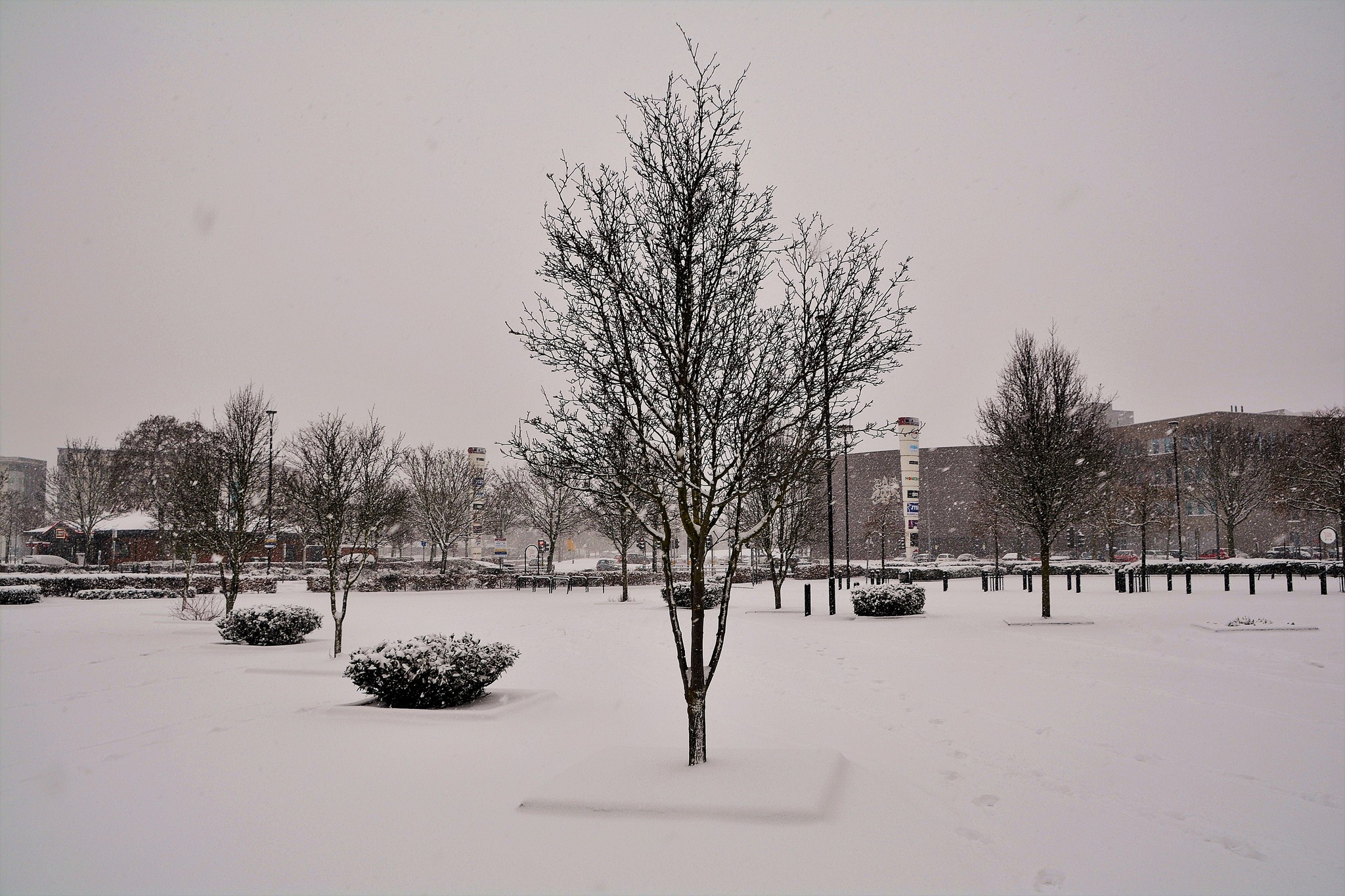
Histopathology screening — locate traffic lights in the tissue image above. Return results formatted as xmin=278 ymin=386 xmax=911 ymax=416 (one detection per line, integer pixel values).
xmin=706 ymin=534 xmax=713 ymax=550
xmin=637 ymin=537 xmax=645 ymax=551
xmin=538 ymin=539 xmax=549 ymax=554
xmin=657 ymin=537 xmax=662 ymax=550
xmin=728 ymin=539 xmax=734 ymax=548
xmin=688 ymin=536 xmax=693 ymax=548
xmin=672 ymin=538 xmax=680 ymax=549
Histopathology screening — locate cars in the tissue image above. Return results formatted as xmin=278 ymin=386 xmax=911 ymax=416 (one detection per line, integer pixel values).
xmin=895 ymin=545 xmax=1294 ymax=564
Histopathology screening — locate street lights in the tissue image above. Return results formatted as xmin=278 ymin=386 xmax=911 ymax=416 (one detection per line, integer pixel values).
xmin=263 ymin=409 xmax=276 ymax=575
xmin=835 ymin=424 xmax=856 ymax=588
xmin=1167 ymin=421 xmax=1184 ymax=562
